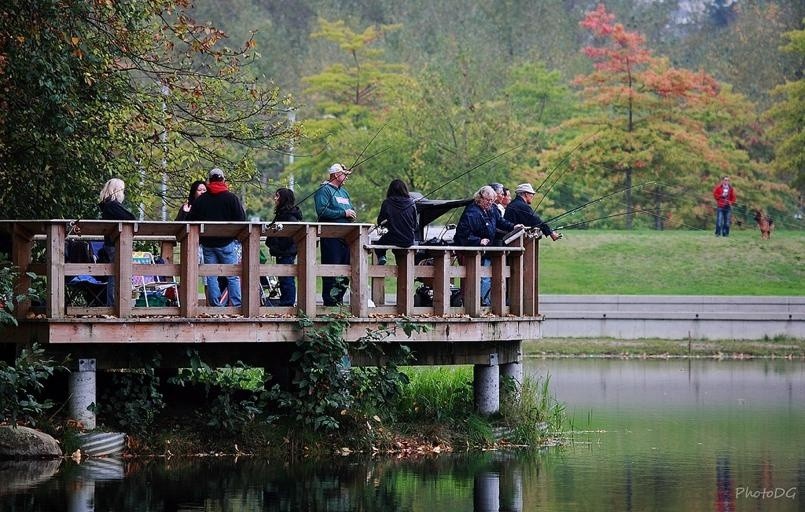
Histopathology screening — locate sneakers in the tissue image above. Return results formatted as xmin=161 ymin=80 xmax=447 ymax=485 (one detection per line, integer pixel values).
xmin=377 ymin=256 xmax=387 ymax=265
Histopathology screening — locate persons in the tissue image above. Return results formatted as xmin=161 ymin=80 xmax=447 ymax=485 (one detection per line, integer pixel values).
xmin=713 ymin=174 xmax=737 ymax=238
xmin=453 ymin=182 xmax=561 ymax=306
xmin=711 ymin=452 xmax=742 ymax=512
xmin=314 ymin=162 xmax=358 ymax=307
xmin=264 ymin=186 xmax=304 ymax=307
xmin=97 ymin=177 xmax=138 ymax=307
xmin=177 ymin=179 xmax=209 ymax=307
xmin=189 ymin=166 xmax=246 ymax=307
xmin=373 ymin=178 xmax=417 ymax=265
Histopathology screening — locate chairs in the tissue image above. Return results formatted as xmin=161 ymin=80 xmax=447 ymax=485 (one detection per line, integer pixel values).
xmin=61 ymin=239 xmax=181 ymax=309
xmin=258 ymin=248 xmax=281 ymax=306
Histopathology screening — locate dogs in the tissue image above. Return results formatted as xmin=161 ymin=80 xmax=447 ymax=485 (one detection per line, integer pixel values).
xmin=754 ymin=209 xmax=776 ymax=239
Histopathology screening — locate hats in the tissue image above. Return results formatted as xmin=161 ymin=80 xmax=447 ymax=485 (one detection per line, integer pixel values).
xmin=514 ymin=182 xmax=537 ymax=194
xmin=329 ymin=163 xmax=352 ymax=175
xmin=209 ymin=168 xmax=225 ymax=179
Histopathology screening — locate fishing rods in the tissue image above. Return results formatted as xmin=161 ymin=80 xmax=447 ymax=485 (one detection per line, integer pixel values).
xmin=520 ymin=129 xmax=675 ymax=233
xmin=270 ymin=122 xmax=384 ymax=298
xmin=367 ymin=143 xmax=527 ymax=235
xmin=272 ymin=146 xmax=393 ymax=224
